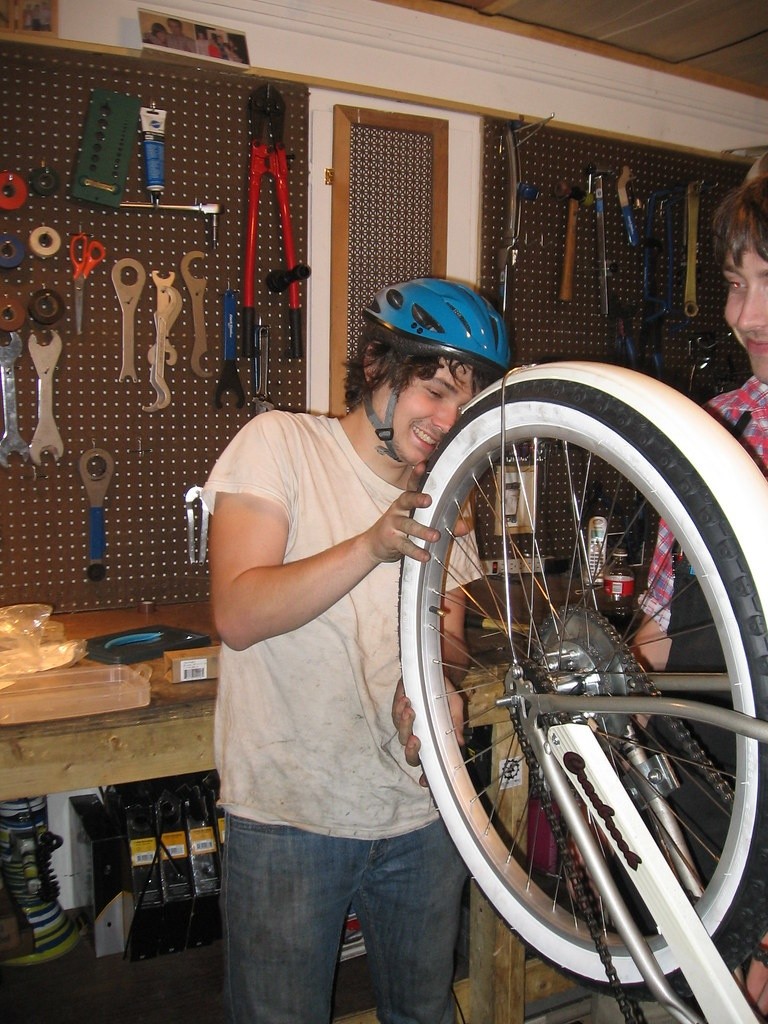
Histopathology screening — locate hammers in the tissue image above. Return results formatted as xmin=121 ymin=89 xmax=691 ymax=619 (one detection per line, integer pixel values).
xmin=551 ymin=181 xmax=594 ymax=302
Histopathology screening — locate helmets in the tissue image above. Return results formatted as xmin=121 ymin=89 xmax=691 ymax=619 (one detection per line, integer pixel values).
xmin=361 ymin=277 xmax=510 ymax=378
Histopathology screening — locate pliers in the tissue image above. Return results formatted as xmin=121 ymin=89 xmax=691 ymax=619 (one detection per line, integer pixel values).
xmin=237 ymin=83 xmax=305 ymax=359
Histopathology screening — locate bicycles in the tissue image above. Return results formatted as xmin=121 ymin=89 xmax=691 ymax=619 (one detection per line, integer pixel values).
xmin=395 ymin=357 xmax=767 ymax=1024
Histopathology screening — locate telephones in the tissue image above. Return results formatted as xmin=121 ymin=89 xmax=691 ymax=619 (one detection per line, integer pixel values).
xmin=581 ymin=514 xmax=608 ymax=585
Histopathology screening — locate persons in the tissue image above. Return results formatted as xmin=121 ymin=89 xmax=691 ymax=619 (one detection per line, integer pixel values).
xmin=200 ymin=276 xmax=508 ymax=1024
xmin=638 ymin=177 xmax=768 ymax=1024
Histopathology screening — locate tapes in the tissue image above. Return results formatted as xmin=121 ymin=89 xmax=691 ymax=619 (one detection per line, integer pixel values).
xmin=0 ymin=299 xmax=25 ymax=331
xmin=29 ymin=226 xmax=62 ymax=259
xmin=0 ymin=172 xmax=27 ymax=210
xmin=0 ymin=234 xmax=25 ymax=269
xmin=30 ymin=166 xmax=60 ymax=195
xmin=28 ymin=288 xmax=64 ymax=326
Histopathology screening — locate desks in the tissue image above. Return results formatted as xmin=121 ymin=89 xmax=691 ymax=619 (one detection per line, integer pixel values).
xmin=0 ymin=601 xmax=530 ymax=1024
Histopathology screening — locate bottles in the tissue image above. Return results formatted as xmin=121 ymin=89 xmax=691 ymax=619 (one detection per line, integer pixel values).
xmin=603 ymin=548 xmax=634 ymax=631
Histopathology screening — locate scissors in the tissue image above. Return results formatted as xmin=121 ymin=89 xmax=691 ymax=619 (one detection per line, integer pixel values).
xmin=69 ymin=232 xmax=107 ymax=335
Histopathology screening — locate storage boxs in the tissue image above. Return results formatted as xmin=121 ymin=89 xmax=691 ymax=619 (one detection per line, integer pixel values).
xmin=0 ymin=663 xmax=153 ymax=725
xmin=202 ymin=789 xmax=226 ymax=861
xmin=179 ymin=792 xmax=222 ymax=950
xmin=150 ymin=792 xmax=194 ymax=955
xmin=103 ymin=795 xmax=164 ymax=963
xmin=164 ymin=647 xmax=221 ymax=683
xmin=69 ymin=794 xmax=125 ymax=959
